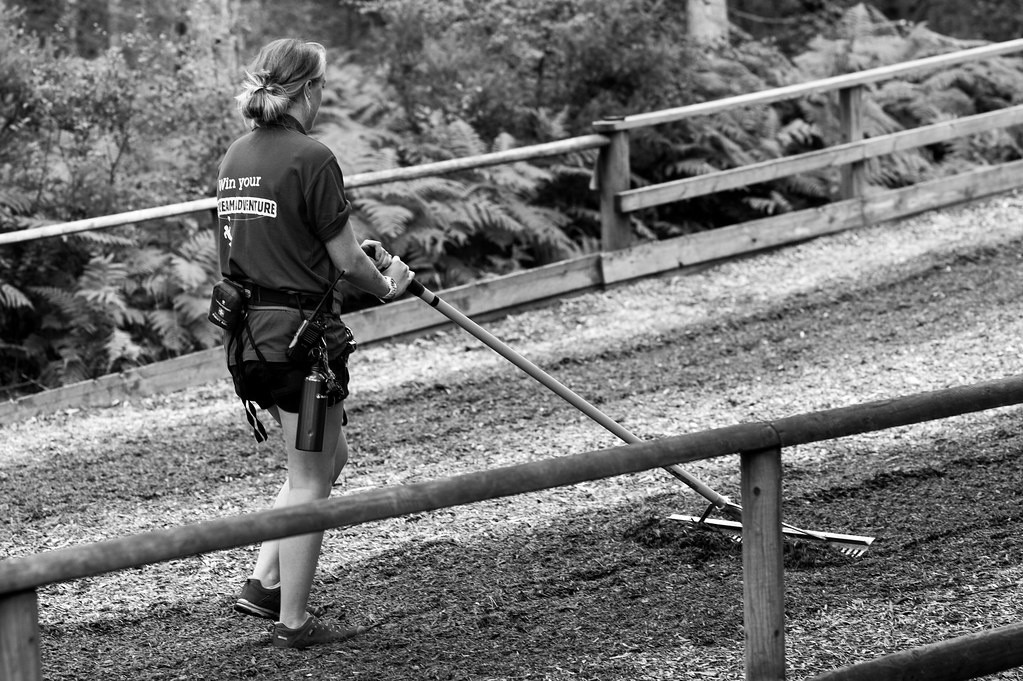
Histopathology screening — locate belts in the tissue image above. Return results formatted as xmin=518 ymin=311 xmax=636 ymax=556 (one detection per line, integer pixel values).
xmin=245 ymin=286 xmax=341 ymax=316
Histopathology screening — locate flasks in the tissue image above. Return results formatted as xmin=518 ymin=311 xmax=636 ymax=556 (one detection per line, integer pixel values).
xmin=295 ymin=348 xmax=328 ymax=452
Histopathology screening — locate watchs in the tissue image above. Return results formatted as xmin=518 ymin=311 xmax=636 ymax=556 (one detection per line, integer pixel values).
xmin=379 ymin=275 xmax=396 ymax=304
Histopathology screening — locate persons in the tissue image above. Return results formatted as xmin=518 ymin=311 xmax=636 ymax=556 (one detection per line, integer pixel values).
xmin=216 ymin=38 xmax=414 ymax=650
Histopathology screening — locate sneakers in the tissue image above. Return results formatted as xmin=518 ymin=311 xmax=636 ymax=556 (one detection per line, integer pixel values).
xmin=275 ymin=614 xmax=355 ymax=650
xmin=234 ymin=578 xmax=283 ymax=619
